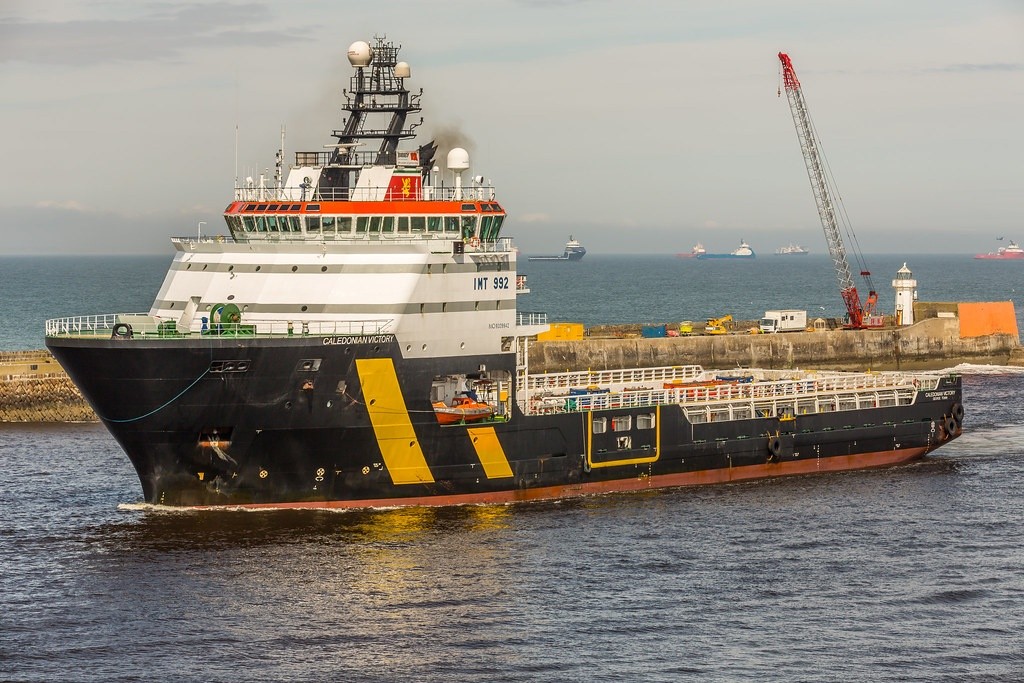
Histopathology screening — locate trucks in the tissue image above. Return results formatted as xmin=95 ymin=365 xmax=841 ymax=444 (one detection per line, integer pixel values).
xmin=757 ymin=309 xmax=807 ymax=333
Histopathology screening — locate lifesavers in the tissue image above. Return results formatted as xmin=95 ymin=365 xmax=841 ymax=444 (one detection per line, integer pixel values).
xmin=517 ymin=277 xmax=524 ymax=288
xmin=768 ymin=437 xmax=784 ymax=457
xmin=470 ymin=236 xmax=480 ymax=247
xmin=945 ymin=418 xmax=957 ymax=437
xmin=113 ymin=322 xmax=130 ymax=338
xmin=952 ymin=403 xmax=964 ymax=422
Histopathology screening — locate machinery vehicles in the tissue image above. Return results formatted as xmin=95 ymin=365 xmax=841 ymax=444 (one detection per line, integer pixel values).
xmin=775 ymin=50 xmax=885 ymax=330
xmin=702 ymin=315 xmax=735 ymax=335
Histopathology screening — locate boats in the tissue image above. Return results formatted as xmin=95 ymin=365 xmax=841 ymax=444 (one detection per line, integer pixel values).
xmin=44 ymin=36 xmax=965 ymax=515
xmin=527 ymin=235 xmax=586 ymax=261
xmin=697 ymin=238 xmax=756 ymax=260
xmin=436 ymin=398 xmax=492 ymax=425
xmin=676 ymin=247 xmax=705 ymax=259
xmin=974 ymin=237 xmax=1024 ymax=261
xmin=774 ymin=244 xmax=808 ymax=256
